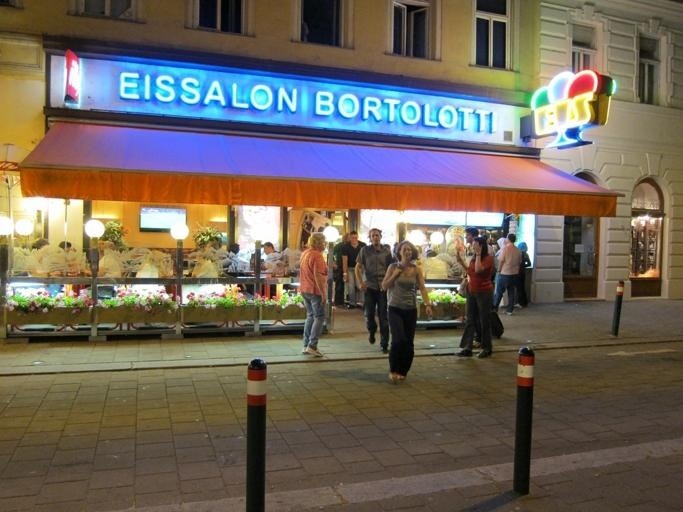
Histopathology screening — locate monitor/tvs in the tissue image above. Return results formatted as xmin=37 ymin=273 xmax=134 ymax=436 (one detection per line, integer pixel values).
xmin=139 ymin=205 xmax=186 ymax=232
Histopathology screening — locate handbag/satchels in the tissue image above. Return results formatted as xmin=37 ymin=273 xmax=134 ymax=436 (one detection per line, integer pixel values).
xmin=458 ymin=277 xmax=469 ymax=298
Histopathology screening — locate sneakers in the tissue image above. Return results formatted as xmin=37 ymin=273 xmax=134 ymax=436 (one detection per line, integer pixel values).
xmin=369 ymin=331 xmax=376 ymax=344
xmin=380 ymin=346 xmax=388 ymax=353
xmin=499 ymin=303 xmax=522 ymax=315
xmin=302 ymin=344 xmax=323 ymax=357
xmin=456 ymin=339 xmax=492 ymax=358
xmin=389 ymin=373 xmax=404 ymax=382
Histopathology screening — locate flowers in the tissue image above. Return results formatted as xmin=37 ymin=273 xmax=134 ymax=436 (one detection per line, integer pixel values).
xmin=192 ymin=223 xmax=222 ymax=246
xmin=99 ymin=221 xmax=128 ymax=243
xmin=416 ymin=288 xmax=466 ymax=307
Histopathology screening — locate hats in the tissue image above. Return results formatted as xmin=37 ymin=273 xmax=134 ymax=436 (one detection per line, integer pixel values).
xmin=261 ymin=242 xmax=273 ymax=247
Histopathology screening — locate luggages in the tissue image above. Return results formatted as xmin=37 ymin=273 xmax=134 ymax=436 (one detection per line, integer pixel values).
xmin=488 ymin=310 xmax=504 ymax=339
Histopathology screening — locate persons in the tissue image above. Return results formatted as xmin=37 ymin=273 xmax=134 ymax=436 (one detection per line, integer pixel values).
xmin=26 ymin=238 xmax=144 ymax=279
xmin=465 ymin=227 xmax=495 ymax=348
xmin=456 ymin=238 xmax=494 ymax=358
xmin=381 ymin=240 xmax=433 ymax=383
xmin=189 ymin=231 xmax=531 ymax=315
xmin=354 ymin=228 xmax=391 ymax=354
xmin=298 ymin=233 xmax=328 ymax=358
